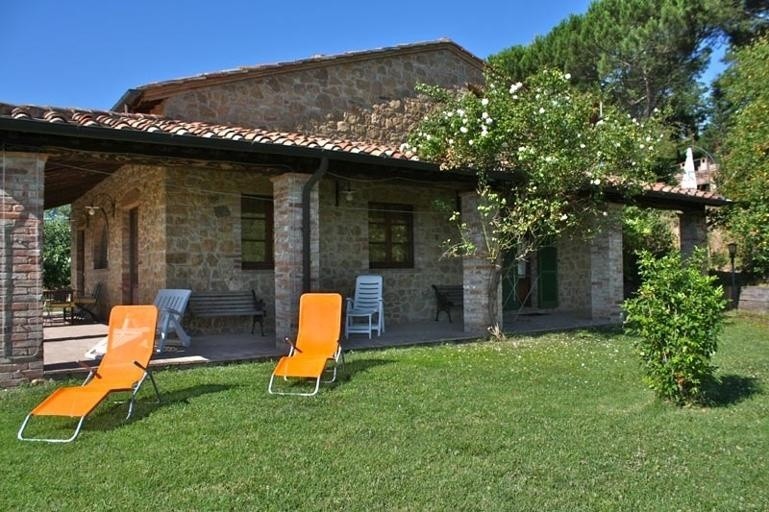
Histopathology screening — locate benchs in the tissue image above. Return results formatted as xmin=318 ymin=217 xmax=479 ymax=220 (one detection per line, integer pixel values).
xmin=431 ymin=284 xmax=464 ymax=324
xmin=185 ymin=291 xmax=268 ymax=337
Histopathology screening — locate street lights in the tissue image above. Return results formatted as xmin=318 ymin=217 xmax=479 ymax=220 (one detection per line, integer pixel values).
xmin=726 ymin=242 xmax=738 ymax=309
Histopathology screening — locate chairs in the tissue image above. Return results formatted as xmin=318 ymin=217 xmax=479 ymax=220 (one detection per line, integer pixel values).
xmin=345 ymin=275 xmax=386 ymax=340
xmin=16 ymin=304 xmax=160 ymax=444
xmin=85 ymin=287 xmax=192 ymax=359
xmin=267 ymin=291 xmax=346 ymax=398
xmin=41 ymin=282 xmax=103 ymax=323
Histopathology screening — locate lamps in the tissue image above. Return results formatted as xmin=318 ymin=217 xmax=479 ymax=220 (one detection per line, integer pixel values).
xmin=85 ymin=192 xmax=116 ymax=229
xmin=334 ymin=178 xmax=356 ymax=206
